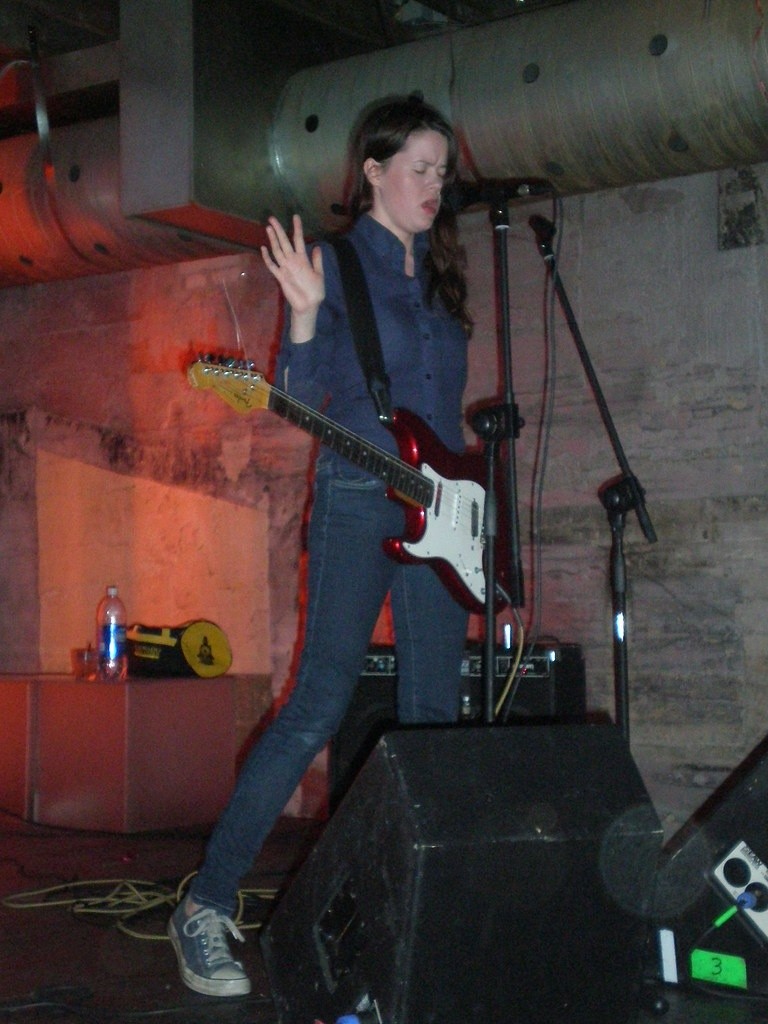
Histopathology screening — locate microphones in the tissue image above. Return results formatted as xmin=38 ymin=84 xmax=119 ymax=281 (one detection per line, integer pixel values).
xmin=440 ymin=178 xmax=548 ymax=211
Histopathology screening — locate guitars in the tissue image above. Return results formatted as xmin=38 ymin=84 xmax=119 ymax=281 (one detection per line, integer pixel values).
xmin=188 ymin=352 xmax=508 ymax=614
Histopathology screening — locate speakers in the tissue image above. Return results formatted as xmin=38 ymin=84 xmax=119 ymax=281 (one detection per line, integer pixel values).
xmin=646 ymin=735 xmax=768 ymax=1024
xmin=260 ymin=722 xmax=666 ymax=1024
xmin=327 ymin=642 xmax=584 ymax=828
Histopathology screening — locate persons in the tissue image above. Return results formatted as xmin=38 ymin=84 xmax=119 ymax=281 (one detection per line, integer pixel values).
xmin=168 ymin=102 xmax=469 ymax=996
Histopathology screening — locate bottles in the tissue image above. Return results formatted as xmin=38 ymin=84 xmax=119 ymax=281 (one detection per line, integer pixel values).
xmin=97 ymin=587 xmax=127 ymax=684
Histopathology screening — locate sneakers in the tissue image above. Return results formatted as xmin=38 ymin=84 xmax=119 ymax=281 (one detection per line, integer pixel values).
xmin=166 ymin=895 xmax=252 ymax=997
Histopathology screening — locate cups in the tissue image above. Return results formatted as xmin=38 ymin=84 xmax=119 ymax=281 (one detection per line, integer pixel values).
xmin=72 ymin=649 xmax=96 ymax=680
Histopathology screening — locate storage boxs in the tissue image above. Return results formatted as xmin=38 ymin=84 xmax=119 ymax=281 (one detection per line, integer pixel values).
xmin=0 ymin=673 xmax=274 ymax=837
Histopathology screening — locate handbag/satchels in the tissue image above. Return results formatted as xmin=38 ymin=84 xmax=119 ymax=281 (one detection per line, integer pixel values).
xmin=122 ymin=616 xmax=232 ymax=681
